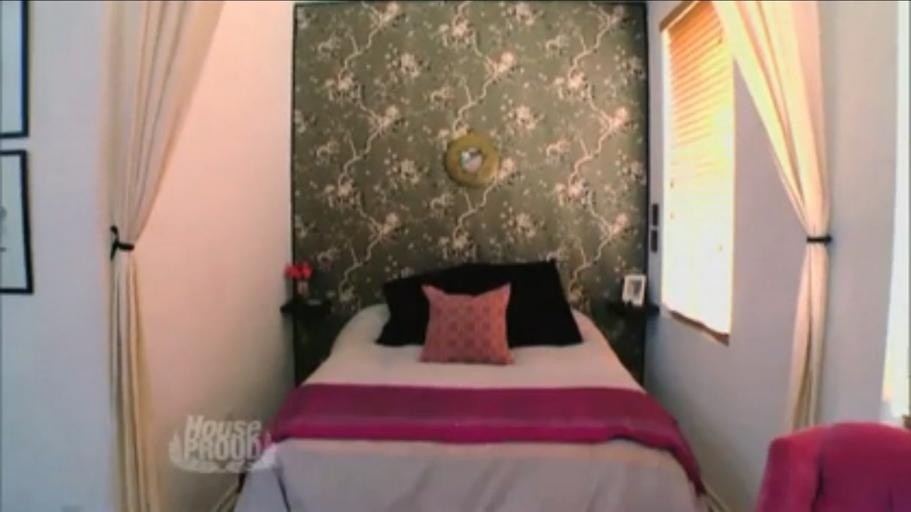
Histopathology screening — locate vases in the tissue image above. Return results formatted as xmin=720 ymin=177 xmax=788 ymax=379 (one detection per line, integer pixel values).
xmin=297 ymin=280 xmax=310 ymax=300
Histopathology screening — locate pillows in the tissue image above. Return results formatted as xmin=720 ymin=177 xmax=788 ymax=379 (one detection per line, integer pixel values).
xmin=376 ymin=258 xmax=582 ymax=364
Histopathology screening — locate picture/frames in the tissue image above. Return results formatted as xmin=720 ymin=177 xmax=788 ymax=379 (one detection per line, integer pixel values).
xmin=0 ymin=0 xmax=28 ymax=138
xmin=621 ymin=275 xmax=646 ymax=306
xmin=0 ymin=150 xmax=33 ymax=294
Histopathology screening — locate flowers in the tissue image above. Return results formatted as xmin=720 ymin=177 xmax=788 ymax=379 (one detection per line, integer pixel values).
xmin=286 ymin=261 xmax=313 ymax=279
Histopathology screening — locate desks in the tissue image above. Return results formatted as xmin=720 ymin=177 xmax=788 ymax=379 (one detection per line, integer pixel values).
xmin=608 ymin=301 xmax=658 ymax=316
xmin=280 ymin=295 xmax=332 ymax=317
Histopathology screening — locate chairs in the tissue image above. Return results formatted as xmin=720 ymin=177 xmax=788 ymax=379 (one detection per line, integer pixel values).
xmin=758 ymin=424 xmax=911 ymax=512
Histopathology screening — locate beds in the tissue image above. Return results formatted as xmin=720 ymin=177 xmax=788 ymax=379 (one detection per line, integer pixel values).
xmin=234 ymin=303 xmax=705 ymax=512
